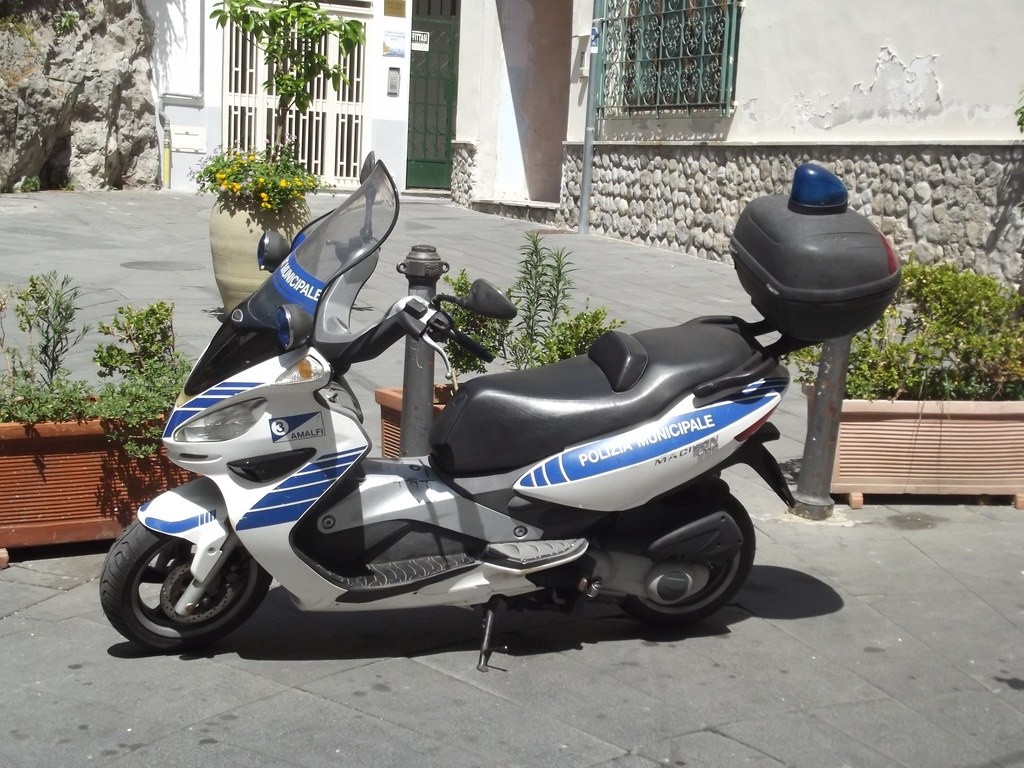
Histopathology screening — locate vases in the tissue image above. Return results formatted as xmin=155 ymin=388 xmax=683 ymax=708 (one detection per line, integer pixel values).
xmin=209 ymin=195 xmax=312 ymax=317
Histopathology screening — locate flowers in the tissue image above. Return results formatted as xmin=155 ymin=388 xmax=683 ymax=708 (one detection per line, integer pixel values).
xmin=185 ymin=132 xmax=321 ymax=219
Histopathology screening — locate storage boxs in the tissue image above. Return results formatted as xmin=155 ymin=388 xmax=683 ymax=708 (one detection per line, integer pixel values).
xmin=728 ymin=193 xmax=902 ymax=340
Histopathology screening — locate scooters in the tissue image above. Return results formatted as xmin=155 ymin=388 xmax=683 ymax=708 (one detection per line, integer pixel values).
xmin=100 ymin=151 xmax=902 ymax=672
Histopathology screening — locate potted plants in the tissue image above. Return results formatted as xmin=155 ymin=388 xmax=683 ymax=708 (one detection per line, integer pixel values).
xmin=778 ymin=250 xmax=1024 ymax=510
xmin=0 ymin=270 xmax=207 ymax=549
xmin=374 ymin=230 xmax=628 ymax=460
